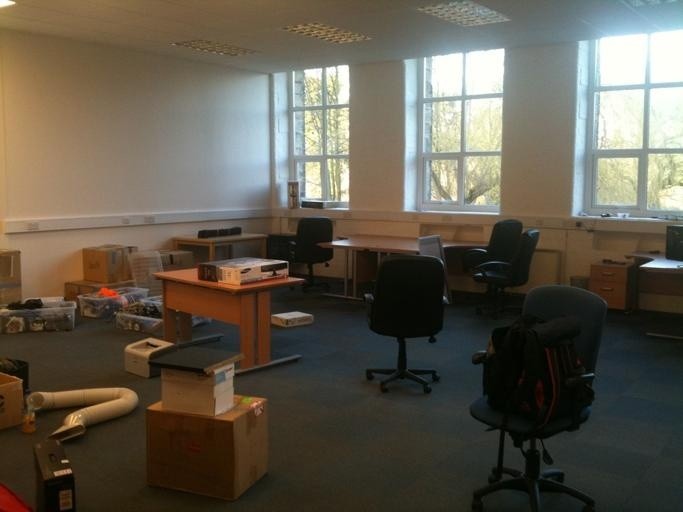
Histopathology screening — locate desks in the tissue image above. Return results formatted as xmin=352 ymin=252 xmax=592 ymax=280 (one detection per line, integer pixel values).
xmin=321 ymin=234 xmax=488 ymax=302
xmin=172 ymin=233 xmax=268 ymax=260
xmin=153 ymin=266 xmax=305 ymax=369
xmin=624 ymin=249 xmax=683 ymax=339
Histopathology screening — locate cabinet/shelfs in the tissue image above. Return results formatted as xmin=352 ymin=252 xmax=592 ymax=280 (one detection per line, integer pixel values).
xmin=592 ymin=258 xmax=636 ymax=315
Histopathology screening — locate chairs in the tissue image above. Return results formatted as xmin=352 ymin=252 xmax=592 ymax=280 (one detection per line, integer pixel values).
xmin=473 ymin=229 xmax=541 ymax=315
xmin=295 ymin=217 xmax=333 ymax=292
xmin=468 ymin=286 xmax=609 ymax=512
xmin=469 ymin=219 xmax=523 ymax=313
xmin=367 ymin=254 xmax=445 ymax=394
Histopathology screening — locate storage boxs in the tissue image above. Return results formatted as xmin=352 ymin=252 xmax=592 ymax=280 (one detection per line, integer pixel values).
xmin=0 ymin=251 xmax=21 ymax=303
xmin=1 ymin=372 xmax=24 ymax=433
xmin=65 ymin=278 xmax=138 ymax=304
xmin=77 ymin=286 xmax=149 ymax=320
xmin=145 ymin=395 xmax=271 ymax=501
xmin=161 ymin=251 xmax=193 ymax=271
xmin=83 ymin=245 xmax=138 ymax=283
xmin=0 ymin=298 xmax=77 ymax=334
xmin=116 ymin=294 xmax=211 ymax=333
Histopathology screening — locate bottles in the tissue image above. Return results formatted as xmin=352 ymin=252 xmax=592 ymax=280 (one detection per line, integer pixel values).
xmin=21 ymin=408 xmax=36 ymax=434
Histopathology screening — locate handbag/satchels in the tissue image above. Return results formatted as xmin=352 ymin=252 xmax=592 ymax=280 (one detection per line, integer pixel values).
xmin=480 ymin=313 xmax=594 ymax=421
